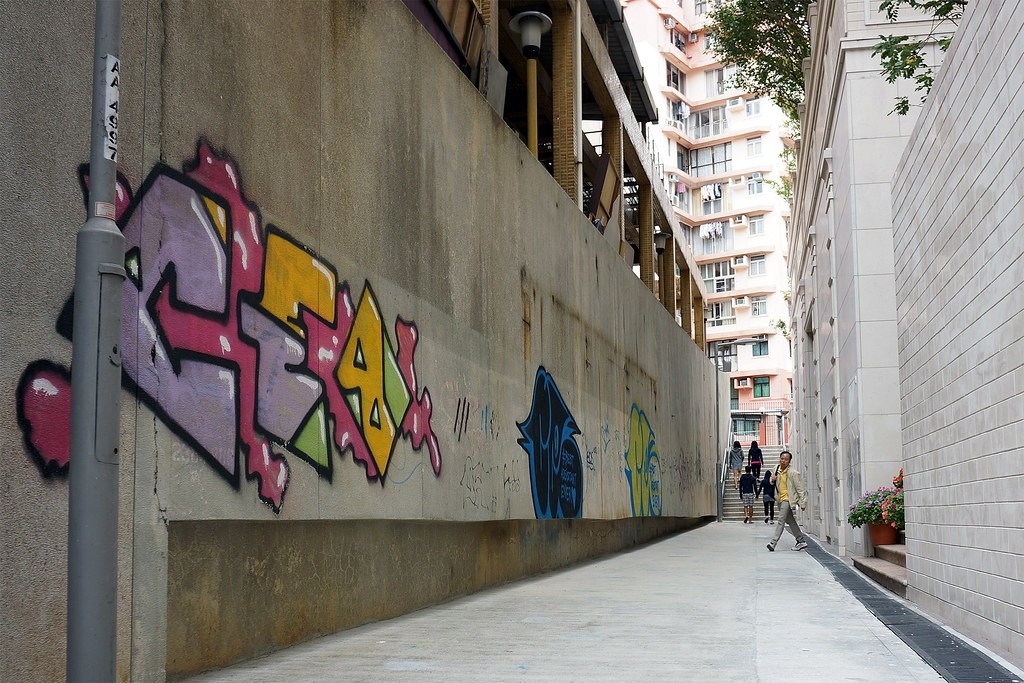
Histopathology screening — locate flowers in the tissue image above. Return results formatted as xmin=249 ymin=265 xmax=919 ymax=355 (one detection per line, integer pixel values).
xmin=847 ymin=469 xmax=905 ymax=529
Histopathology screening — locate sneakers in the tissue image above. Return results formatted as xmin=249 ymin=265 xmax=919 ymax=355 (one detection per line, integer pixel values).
xmin=767 ymin=542 xmax=775 ymax=552
xmin=791 ymin=542 xmax=808 ymax=551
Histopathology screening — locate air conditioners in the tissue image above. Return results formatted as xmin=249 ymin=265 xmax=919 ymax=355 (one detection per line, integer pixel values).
xmin=736 ymin=299 xmax=745 ymax=305
xmin=679 ymin=105 xmax=690 ymax=115
xmin=670 ymin=173 xmax=679 ymax=182
xmin=666 ymin=18 xmax=676 ymax=29
xmin=736 ymin=257 xmax=743 ymax=263
xmin=736 ymin=215 xmax=742 ymax=221
xmin=752 ymin=172 xmax=762 ymax=180
xmin=690 ymin=34 xmax=697 ymax=40
xmin=730 ymin=98 xmax=740 ymax=105
xmin=739 ymin=380 xmax=747 ymax=386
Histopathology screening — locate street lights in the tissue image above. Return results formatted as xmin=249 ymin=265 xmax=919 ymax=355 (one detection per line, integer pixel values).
xmin=507 ymin=10 xmax=553 ymax=161
xmin=713 ymin=335 xmax=769 ymax=523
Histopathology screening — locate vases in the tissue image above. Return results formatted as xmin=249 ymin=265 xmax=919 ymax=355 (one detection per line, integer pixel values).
xmin=869 ymin=519 xmax=898 ymax=546
xmin=897 ymin=486 xmax=903 ymax=489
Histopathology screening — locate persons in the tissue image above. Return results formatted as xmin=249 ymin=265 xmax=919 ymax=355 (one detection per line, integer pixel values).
xmin=729 ymin=441 xmax=744 ymax=490
xmin=738 ymin=466 xmax=759 ymax=523
xmin=748 ymin=441 xmax=763 ymax=483
xmin=755 ymin=471 xmax=779 ymax=526
xmin=766 ymin=452 xmax=809 ymax=552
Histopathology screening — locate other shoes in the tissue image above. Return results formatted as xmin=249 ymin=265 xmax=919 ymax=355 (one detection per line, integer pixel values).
xmin=765 ymin=516 xmax=769 ymax=523
xmin=770 ymin=520 xmax=775 ymax=524
xmin=744 ymin=517 xmax=749 ymax=523
xmin=757 ymin=479 xmax=761 ymax=483
